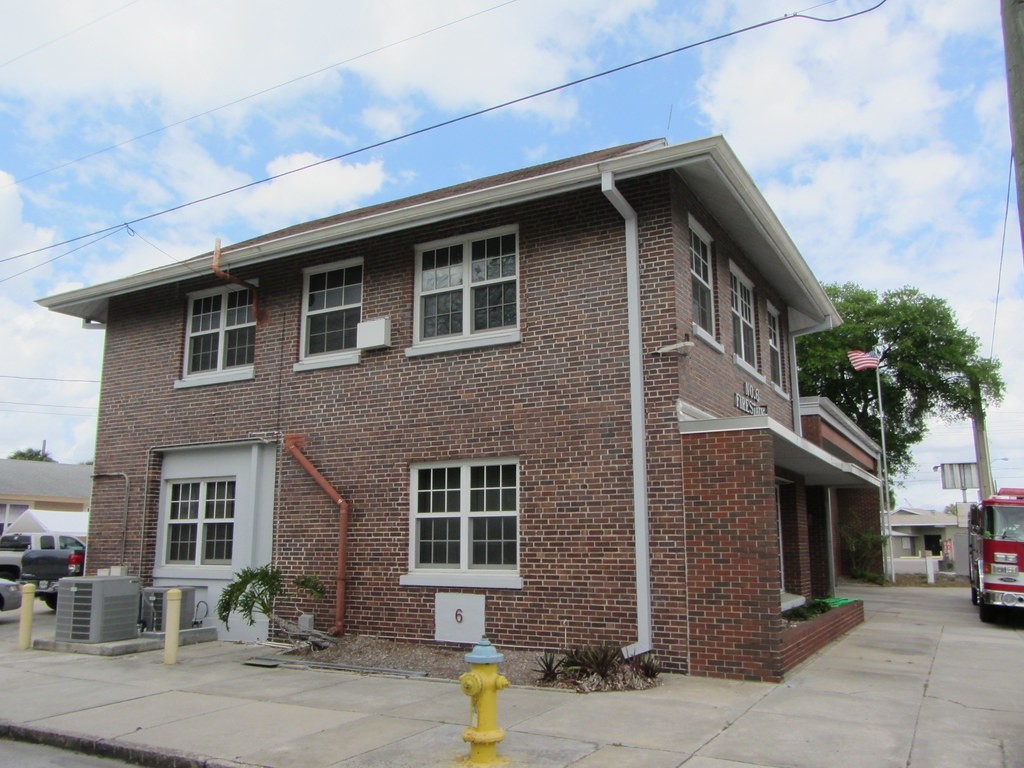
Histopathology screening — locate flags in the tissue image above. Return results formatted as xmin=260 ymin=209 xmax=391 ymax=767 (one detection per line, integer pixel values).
xmin=847 ymin=350 xmax=879 ymax=371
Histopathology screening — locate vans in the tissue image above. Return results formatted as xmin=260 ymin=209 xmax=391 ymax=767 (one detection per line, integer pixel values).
xmin=0 ymin=532 xmax=86 ymax=582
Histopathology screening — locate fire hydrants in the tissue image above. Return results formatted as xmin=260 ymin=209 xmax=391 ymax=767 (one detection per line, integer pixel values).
xmin=459 ymin=638 xmax=512 ymax=764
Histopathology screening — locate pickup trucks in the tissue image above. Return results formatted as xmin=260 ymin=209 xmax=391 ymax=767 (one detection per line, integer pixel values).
xmin=19 ymin=549 xmax=85 ymax=610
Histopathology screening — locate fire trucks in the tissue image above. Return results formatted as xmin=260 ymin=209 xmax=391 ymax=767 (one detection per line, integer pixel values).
xmin=968 ymin=487 xmax=1024 ymax=622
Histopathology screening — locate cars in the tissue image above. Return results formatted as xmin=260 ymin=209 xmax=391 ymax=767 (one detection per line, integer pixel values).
xmin=0 ymin=579 xmax=22 ymax=611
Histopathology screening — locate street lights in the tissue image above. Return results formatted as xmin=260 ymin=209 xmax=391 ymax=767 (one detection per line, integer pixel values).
xmin=991 ymin=457 xmax=1009 ymax=462
